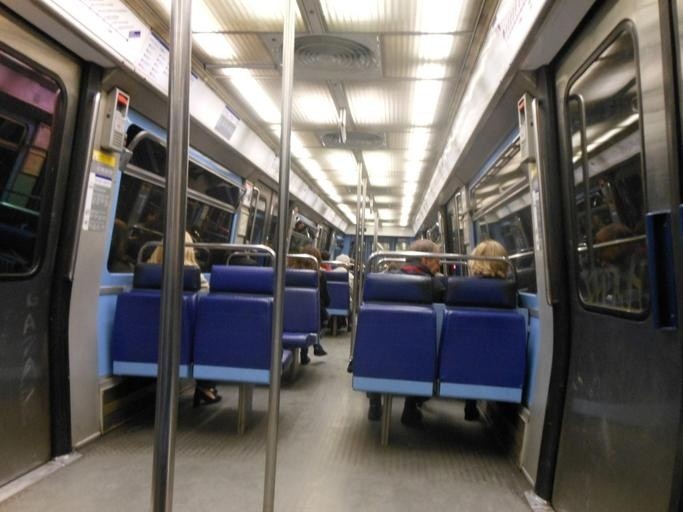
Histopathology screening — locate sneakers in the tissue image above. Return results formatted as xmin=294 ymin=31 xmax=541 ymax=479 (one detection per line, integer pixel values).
xmin=300 ymin=353 xmax=310 ymax=363
xmin=314 ymin=346 xmax=327 ymax=355
xmin=464 ymin=408 xmax=479 ymax=420
xmin=402 ymin=408 xmax=422 ymax=422
xmin=368 ymin=402 xmax=380 ymax=419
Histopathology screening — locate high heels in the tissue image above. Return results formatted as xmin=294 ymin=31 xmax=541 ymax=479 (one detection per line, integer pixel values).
xmin=193 ymin=386 xmax=222 ymax=404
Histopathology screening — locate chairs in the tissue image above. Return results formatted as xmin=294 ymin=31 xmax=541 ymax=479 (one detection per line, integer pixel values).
xmin=577 ymin=261 xmax=615 ymax=303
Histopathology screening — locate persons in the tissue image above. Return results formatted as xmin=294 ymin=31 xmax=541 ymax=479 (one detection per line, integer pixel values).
xmin=223 ymin=237 xmax=260 ymax=268
xmin=286 ymin=243 xmax=328 ymax=366
xmin=363 ymin=238 xmax=446 ymax=425
xmin=383 ymin=260 xmax=398 ymax=272
xmin=578 ymin=222 xmax=640 ymax=311
xmin=318 ymin=249 xmax=365 ymax=336
xmin=109 ymin=216 xmax=138 ymax=275
xmin=443 ymin=239 xmax=512 ymax=423
xmin=144 ymin=224 xmax=223 ymax=408
